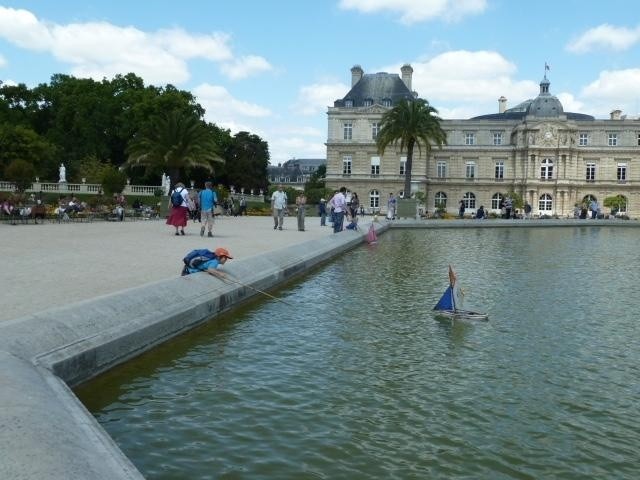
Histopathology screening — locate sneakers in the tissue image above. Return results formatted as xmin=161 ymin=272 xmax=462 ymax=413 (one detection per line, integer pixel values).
xmin=175 ymin=229 xmax=185 ymax=235
xmin=200 ymin=227 xmax=213 ymax=237
xmin=273 ymin=225 xmax=283 ymax=231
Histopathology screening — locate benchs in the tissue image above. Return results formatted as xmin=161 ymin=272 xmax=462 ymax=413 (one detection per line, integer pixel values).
xmin=0 ymin=209 xmax=142 ymax=225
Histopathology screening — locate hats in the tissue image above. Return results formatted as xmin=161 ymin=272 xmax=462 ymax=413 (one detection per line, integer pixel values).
xmin=215 ymin=248 xmax=232 ymax=259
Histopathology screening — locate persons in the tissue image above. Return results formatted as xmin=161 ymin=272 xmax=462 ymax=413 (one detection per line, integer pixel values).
xmin=181 ymin=248 xmax=234 ymax=278
xmin=165 ymin=182 xmax=195 ymax=235
xmin=222 ymin=184 xmax=365 ymax=234
xmin=3 ymin=190 xmax=126 ymax=222
xmin=198 ymin=181 xmax=218 ymax=236
xmin=458 ymin=196 xmax=598 ymax=219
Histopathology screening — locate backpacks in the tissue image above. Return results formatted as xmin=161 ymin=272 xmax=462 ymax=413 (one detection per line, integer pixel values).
xmin=184 ymin=249 xmax=215 ymax=269
xmin=171 ymin=188 xmax=186 ymax=207
